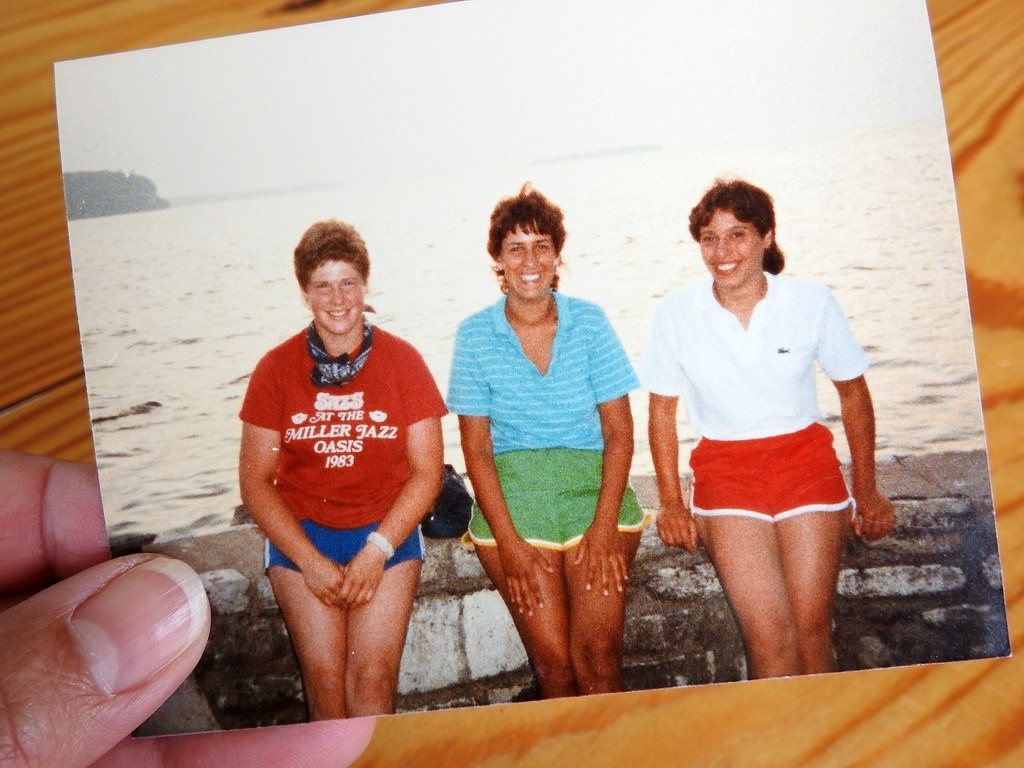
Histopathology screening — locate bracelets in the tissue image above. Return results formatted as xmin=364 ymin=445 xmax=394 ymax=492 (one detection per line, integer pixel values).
xmin=365 ymin=531 xmax=395 ymax=562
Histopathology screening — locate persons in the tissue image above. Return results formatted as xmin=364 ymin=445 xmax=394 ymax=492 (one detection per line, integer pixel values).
xmin=648 ymin=179 xmax=897 ymax=681
xmin=446 ymin=183 xmax=646 ymax=701
xmin=239 ymin=222 xmax=449 ymax=721
xmin=0 ymin=449 xmax=378 ymax=768
